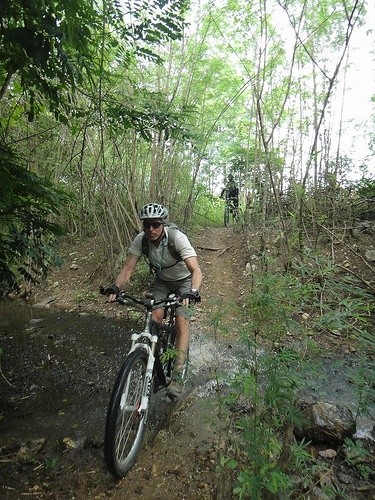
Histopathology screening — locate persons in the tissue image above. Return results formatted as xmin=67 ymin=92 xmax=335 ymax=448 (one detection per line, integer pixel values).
xmin=106 ymin=203 xmax=202 ymax=398
xmin=221 ymin=175 xmax=239 ymax=218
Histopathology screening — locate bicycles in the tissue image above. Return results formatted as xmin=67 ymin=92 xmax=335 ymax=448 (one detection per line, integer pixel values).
xmin=218 ymin=194 xmax=245 ymax=227
xmin=97 ymin=284 xmax=203 ymax=478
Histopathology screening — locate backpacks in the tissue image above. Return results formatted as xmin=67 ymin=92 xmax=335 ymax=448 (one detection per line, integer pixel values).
xmin=142 ymin=223 xmax=196 ymax=260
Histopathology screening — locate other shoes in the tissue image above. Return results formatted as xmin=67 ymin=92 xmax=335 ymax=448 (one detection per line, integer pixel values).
xmin=168 ymin=380 xmax=182 ymax=394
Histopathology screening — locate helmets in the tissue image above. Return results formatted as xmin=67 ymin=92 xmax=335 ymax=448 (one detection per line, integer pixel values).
xmin=140 ymin=203 xmax=168 ymax=220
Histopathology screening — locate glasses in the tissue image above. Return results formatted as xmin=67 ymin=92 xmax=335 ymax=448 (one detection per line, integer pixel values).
xmin=143 ymin=222 xmax=164 ymax=228
